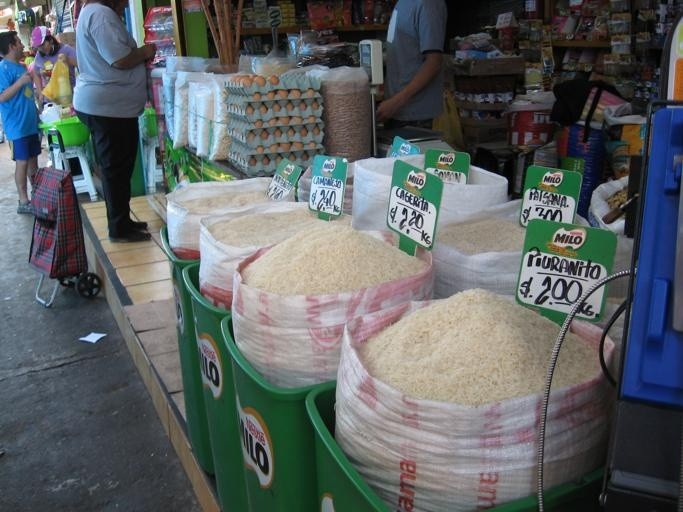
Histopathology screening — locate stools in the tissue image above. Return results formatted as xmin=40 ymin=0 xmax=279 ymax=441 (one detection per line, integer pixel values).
xmin=47 ymin=145 xmax=98 ymax=202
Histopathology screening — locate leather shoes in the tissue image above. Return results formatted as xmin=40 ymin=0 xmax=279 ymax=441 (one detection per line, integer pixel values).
xmin=109 ymin=221 xmax=151 ymax=242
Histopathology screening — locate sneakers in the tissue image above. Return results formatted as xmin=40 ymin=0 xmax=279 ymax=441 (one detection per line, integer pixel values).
xmin=17 ymin=200 xmax=32 ymax=214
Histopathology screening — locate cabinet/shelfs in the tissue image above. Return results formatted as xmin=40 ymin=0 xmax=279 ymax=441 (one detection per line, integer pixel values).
xmin=520 ymin=0 xmax=636 ymax=101
xmin=451 ymin=74 xmax=526 ymax=166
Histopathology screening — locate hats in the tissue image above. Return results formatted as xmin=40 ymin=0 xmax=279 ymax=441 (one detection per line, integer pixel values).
xmin=31 ymin=26 xmax=51 ymax=47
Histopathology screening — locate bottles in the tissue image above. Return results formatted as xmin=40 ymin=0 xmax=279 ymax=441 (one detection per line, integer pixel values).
xmin=143 ymin=101 xmax=158 ymax=137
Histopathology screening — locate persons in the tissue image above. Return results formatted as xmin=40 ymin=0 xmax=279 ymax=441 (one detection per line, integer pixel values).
xmin=0 ymin=31 xmax=42 ymax=214
xmin=375 ymin=0 xmax=448 ymax=129
xmin=31 ymin=25 xmax=77 ymax=115
xmin=72 ymin=0 xmax=157 ymax=243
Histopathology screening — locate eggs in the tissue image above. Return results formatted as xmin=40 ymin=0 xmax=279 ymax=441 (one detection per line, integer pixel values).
xmin=225 ymin=73 xmax=327 ymax=168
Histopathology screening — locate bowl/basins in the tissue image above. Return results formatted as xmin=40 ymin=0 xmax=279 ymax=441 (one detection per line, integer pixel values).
xmin=37 ymin=116 xmax=90 ymax=148
